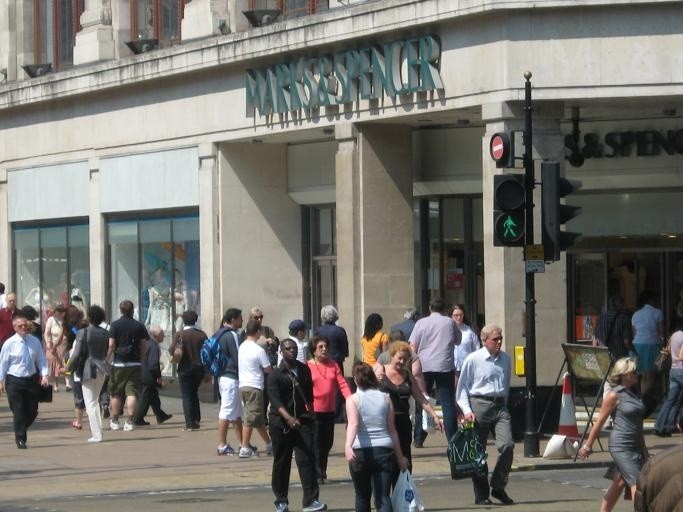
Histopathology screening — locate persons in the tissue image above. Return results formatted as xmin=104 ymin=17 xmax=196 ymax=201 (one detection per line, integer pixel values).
xmin=1 ymin=285 xmax=171 ymax=449
xmin=344 ymin=297 xmax=515 ymax=510
xmin=24 ymin=270 xmax=88 ymax=325
xmin=213 ymin=305 xmax=351 ymax=510
xmin=138 ymin=266 xmax=191 ymax=377
xmin=170 ymin=311 xmax=210 ymax=431
xmin=578 ymin=289 xmax=682 ymax=512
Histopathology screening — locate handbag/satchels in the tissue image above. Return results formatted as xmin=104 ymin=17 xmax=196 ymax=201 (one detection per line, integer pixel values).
xmin=374 ymin=346 xmax=381 ymax=359
xmin=75 ymin=354 xmax=86 ymax=377
xmin=268 ymin=349 xmax=278 ymax=366
xmin=289 ymin=412 xmax=318 ymax=456
xmin=422 ymin=395 xmax=437 ymax=433
xmin=39 ymin=384 xmax=52 ymax=402
xmin=654 ymin=349 xmax=672 ymax=371
xmin=169 ymin=344 xmax=182 ymax=364
xmin=447 ymin=421 xmax=485 ymax=479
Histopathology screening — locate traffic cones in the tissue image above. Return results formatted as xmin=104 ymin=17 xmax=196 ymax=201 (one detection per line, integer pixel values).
xmin=557 ymin=373 xmax=580 ymax=442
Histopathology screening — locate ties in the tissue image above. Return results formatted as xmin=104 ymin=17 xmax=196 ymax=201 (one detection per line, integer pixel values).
xmin=22 ymin=337 xmax=32 ymax=371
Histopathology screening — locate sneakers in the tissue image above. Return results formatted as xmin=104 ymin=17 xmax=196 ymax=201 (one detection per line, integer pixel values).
xmin=412 ymin=440 xmax=423 ymax=448
xmin=183 ymin=424 xmax=200 ymax=431
xmin=302 ymin=500 xmax=326 ymax=512
xmin=217 ymin=442 xmax=272 ymax=457
xmin=652 ymin=424 xmax=683 ymax=437
xmin=54 ymin=386 xmax=72 ymax=393
xmin=275 ymin=502 xmax=287 ymax=512
xmin=492 ymin=487 xmax=513 ymax=505
xmin=317 ymin=477 xmax=332 ymax=484
xmin=69 ymin=406 xmax=172 ymax=431
xmin=88 ymin=435 xmax=103 ymax=442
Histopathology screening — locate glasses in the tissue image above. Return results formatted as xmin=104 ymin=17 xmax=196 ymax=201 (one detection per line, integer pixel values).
xmin=254 ymin=315 xmax=263 ymax=319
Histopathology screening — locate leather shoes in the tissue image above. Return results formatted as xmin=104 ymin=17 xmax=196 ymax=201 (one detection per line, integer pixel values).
xmin=477 ymin=498 xmax=490 ymax=505
xmin=17 ymin=440 xmax=26 ymax=448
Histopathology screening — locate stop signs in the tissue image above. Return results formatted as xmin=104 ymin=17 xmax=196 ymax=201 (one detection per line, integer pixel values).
xmin=491 ymin=134 xmax=504 ymax=160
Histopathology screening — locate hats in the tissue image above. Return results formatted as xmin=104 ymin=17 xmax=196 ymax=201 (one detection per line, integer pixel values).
xmin=289 ymin=320 xmax=306 ymax=329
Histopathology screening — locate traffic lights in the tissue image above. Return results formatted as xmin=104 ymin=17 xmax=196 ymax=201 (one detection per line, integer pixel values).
xmin=542 ymin=163 xmax=582 ymax=263
xmin=493 ymin=175 xmax=525 ymax=247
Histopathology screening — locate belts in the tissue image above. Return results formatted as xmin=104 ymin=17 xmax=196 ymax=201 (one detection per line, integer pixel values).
xmin=473 ymin=396 xmax=504 ymax=402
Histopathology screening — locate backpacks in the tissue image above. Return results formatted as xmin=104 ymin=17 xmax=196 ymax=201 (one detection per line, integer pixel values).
xmin=199 ymin=327 xmax=233 ymax=377
